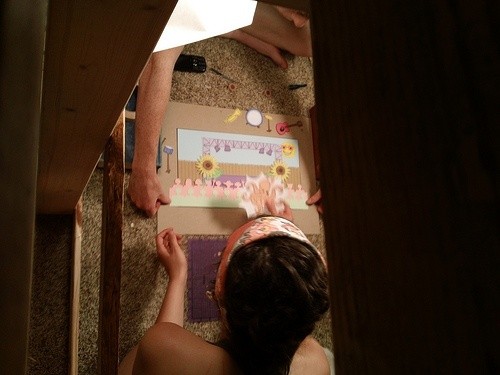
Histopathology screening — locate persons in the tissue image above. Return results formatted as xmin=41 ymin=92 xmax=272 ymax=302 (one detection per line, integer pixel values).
xmin=117 ymin=199 xmax=335 ymax=375
xmin=127 ymin=3 xmax=324 ymax=217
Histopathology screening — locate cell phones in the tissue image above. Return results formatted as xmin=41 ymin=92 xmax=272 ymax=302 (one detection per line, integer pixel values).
xmin=174 ymin=54 xmax=206 ymax=73
